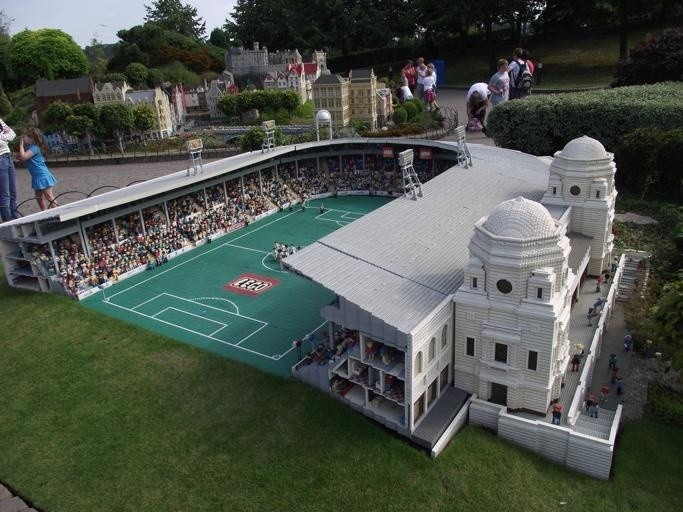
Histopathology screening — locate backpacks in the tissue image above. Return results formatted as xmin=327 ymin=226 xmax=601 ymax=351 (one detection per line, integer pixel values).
xmin=467 ymin=118 xmax=484 ymax=131
xmin=512 ymin=60 xmax=533 ymax=90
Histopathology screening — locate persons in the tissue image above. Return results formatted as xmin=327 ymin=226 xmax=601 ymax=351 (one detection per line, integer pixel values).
xmin=506 ymin=48 xmax=532 ymax=101
xmin=394 ymin=72 xmax=412 ymax=104
xmin=400 ymin=60 xmax=415 ymax=95
xmin=465 ymin=82 xmax=490 ymax=128
xmin=521 ymin=50 xmax=534 ymax=78
xmin=304 ymin=327 xmax=399 ymax=395
xmin=412 ymin=58 xmax=426 ymax=100
xmin=422 ymin=69 xmax=434 ymax=113
xmin=426 ymin=63 xmax=437 ymax=102
xmin=487 ymin=58 xmax=510 ymax=111
xmin=18 ymin=125 xmax=58 ymax=212
xmin=27 ymin=145 xmax=446 ymax=298
xmin=547 ymin=271 xmax=672 ymax=426
xmin=0 ymin=117 xmax=22 ymax=223
